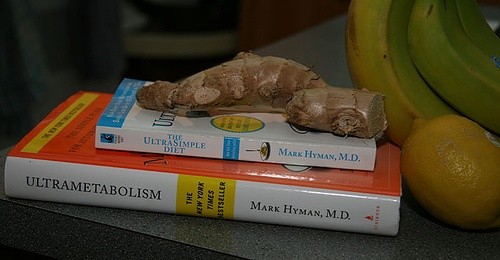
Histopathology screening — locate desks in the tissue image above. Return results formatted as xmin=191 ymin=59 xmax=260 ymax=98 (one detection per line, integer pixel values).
xmin=0 ymin=12 xmax=500 ymax=260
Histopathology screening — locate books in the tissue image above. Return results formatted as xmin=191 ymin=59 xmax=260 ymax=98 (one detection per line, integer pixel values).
xmin=4 ymin=91 xmax=402 ymax=237
xmin=95 ymin=78 xmax=376 ymax=172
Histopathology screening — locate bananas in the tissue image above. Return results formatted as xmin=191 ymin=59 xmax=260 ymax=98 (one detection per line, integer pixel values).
xmin=346 ymin=0 xmax=500 ymax=148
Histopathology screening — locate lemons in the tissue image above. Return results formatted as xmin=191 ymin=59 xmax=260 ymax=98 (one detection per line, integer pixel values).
xmin=402 ymin=114 xmax=500 ymax=229
